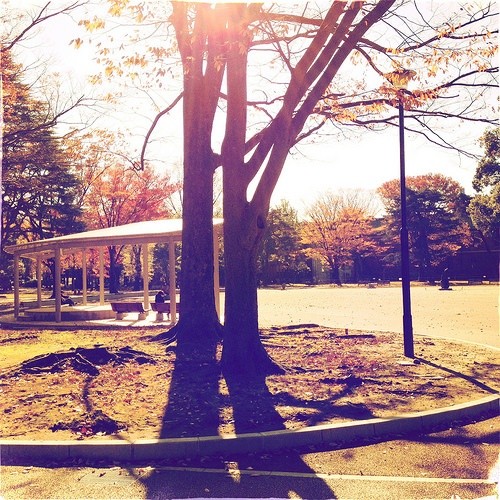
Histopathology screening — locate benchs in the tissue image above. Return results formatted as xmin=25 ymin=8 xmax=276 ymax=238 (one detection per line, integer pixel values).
xmin=150 ymin=302 xmax=180 ymax=322
xmin=111 ymin=302 xmax=151 ymax=320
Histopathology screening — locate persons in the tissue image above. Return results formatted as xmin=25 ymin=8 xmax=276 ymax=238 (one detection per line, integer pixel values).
xmin=155 ymin=291 xmax=165 ymax=314
xmin=61 ymin=291 xmax=75 ymax=306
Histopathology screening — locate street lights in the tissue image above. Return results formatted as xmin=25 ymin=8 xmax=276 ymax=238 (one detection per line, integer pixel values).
xmin=389 ymin=69 xmax=419 ymax=357
